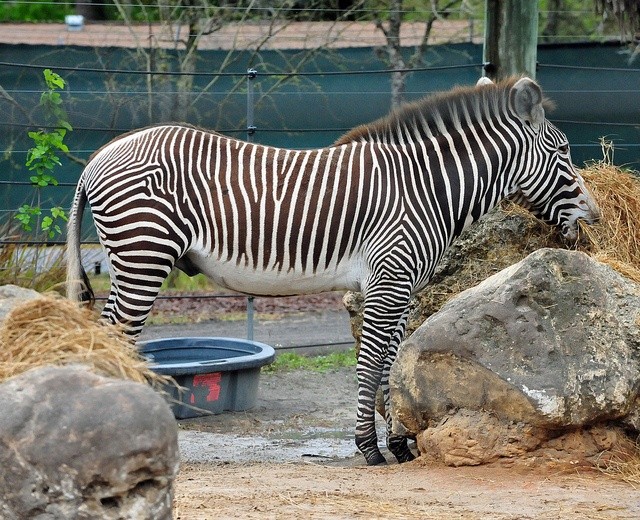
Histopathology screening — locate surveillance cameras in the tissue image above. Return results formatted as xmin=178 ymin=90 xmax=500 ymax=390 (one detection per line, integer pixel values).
xmin=65 ymin=15 xmax=84 ymax=31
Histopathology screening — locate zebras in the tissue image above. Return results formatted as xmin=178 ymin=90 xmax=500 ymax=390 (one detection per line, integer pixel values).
xmin=65 ymin=73 xmax=602 ymax=465
